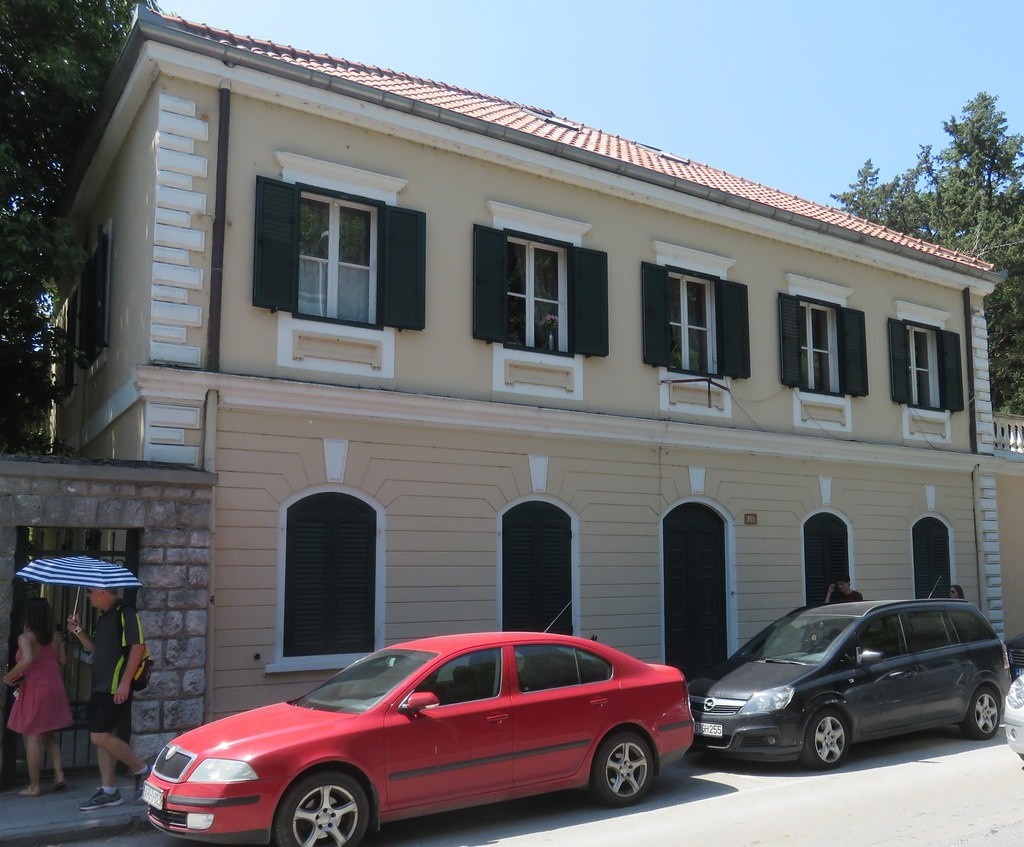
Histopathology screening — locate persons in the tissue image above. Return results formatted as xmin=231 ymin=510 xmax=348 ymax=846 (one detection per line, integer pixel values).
xmin=948 ymin=584 xmax=965 ymax=599
xmin=821 ymin=573 xmax=864 ymax=605
xmin=3 ymin=598 xmax=73 ymax=796
xmin=66 ymin=586 xmax=154 ymax=811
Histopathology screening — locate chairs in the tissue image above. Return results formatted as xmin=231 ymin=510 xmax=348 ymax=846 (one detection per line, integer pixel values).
xmin=453 ymin=666 xmax=473 ymax=700
xmin=547 ymin=657 xmax=584 ymax=689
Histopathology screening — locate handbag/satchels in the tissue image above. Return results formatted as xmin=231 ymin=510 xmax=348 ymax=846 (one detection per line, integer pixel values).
xmin=116 ymin=605 xmax=154 ymax=691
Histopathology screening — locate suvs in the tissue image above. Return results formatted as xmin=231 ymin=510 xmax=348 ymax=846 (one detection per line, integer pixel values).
xmin=687 ymin=600 xmax=1015 ymax=772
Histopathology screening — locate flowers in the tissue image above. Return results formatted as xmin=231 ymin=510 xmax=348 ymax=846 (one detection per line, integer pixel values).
xmin=540 ymin=314 xmax=558 ymax=331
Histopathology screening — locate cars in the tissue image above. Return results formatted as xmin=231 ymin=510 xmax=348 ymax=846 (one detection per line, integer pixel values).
xmin=1003 ymin=633 xmax=1024 ymax=676
xmin=1004 ymin=675 xmax=1024 ymax=759
xmin=140 ymin=631 xmax=698 ymax=847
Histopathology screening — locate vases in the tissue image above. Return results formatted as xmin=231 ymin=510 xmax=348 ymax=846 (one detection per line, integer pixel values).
xmin=549 ymin=331 xmax=554 ymax=350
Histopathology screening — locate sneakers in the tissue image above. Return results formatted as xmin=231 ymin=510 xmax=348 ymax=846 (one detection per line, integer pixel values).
xmin=80 ymin=788 xmax=123 ymax=810
xmin=133 ymin=764 xmax=152 ymax=803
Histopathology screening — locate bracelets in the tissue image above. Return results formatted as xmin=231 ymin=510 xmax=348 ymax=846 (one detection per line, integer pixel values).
xmin=3 ymin=679 xmax=9 ymax=685
xmin=73 ymin=625 xmax=83 ymax=635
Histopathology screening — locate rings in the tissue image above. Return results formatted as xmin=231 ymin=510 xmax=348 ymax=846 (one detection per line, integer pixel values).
xmin=122 ymin=697 xmax=124 ymax=700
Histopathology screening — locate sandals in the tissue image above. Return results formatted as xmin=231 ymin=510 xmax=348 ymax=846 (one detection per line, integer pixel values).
xmin=19 ymin=786 xmax=41 ymax=795
xmin=55 ymin=775 xmax=65 ymax=784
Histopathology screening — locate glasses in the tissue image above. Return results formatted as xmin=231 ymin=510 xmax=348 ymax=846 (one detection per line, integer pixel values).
xmin=950 ymin=591 xmax=955 ymax=593
xmin=88 ymin=589 xmax=93 ymax=594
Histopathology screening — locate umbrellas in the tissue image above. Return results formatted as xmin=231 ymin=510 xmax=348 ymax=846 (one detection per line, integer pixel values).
xmin=16 ymin=555 xmax=144 ymax=621
